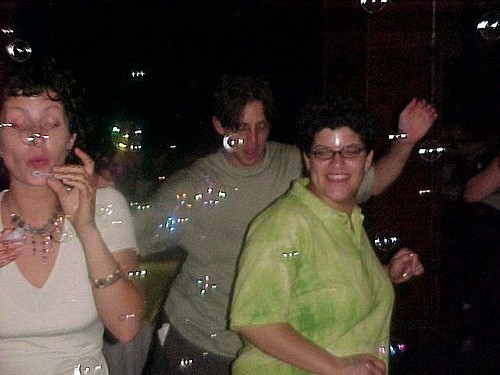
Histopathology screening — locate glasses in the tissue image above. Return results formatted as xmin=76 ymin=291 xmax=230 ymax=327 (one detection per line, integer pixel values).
xmin=309 ymin=145 xmax=367 ymax=161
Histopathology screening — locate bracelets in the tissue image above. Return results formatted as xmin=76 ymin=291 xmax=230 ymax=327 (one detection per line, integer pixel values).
xmin=89 ymin=262 xmax=124 ymax=289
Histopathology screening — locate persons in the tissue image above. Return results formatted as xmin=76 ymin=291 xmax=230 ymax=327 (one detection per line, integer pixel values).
xmin=133 ymin=68 xmax=438 ymax=375
xmin=0 ymin=56 xmax=146 ymax=375
xmin=230 ymin=108 xmax=425 ymax=375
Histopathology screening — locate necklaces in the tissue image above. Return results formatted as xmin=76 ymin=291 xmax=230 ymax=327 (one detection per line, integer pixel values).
xmin=4 ymin=191 xmax=63 ymax=261
